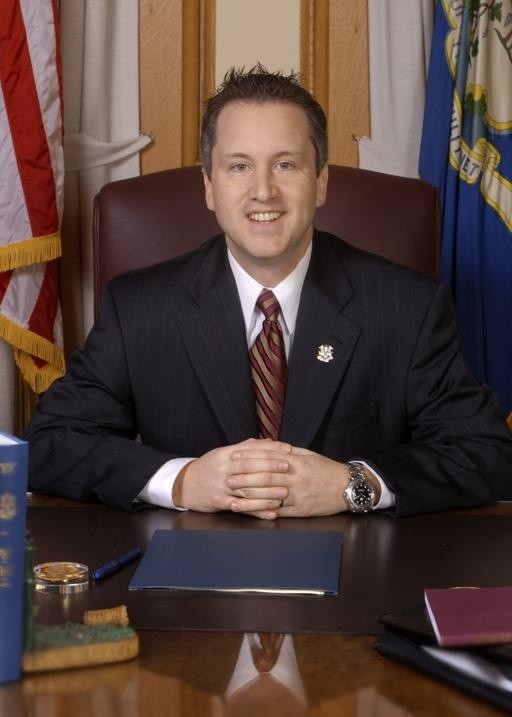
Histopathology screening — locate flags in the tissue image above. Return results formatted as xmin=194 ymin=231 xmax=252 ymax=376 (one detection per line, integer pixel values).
xmin=0 ymin=0 xmax=67 ymax=396
xmin=418 ymin=0 xmax=511 ymax=427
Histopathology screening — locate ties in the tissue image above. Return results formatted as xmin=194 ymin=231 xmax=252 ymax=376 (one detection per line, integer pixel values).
xmin=249 ymin=290 xmax=290 ymax=443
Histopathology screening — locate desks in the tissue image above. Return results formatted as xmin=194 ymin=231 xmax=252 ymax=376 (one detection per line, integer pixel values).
xmin=0 ymin=494 xmax=512 ymax=717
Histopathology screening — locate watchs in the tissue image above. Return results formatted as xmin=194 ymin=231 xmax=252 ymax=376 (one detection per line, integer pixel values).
xmin=342 ymin=458 xmax=376 ymax=514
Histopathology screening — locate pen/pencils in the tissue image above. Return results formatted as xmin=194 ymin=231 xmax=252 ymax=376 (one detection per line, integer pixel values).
xmin=94 ymin=547 xmax=142 ymax=581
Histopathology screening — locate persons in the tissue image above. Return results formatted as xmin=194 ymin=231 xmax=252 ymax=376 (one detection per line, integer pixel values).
xmin=22 ymin=60 xmax=511 ymax=522
xmin=122 ymin=600 xmax=398 ymax=711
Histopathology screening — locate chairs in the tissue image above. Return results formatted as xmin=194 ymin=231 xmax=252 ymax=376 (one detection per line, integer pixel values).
xmin=92 ymin=165 xmax=442 ymax=326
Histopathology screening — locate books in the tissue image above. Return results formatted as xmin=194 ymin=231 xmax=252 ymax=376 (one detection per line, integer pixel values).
xmin=423 ymin=582 xmax=512 ymax=648
xmin=370 ymin=594 xmax=511 ymax=715
xmin=0 ymin=431 xmax=34 ymax=686
